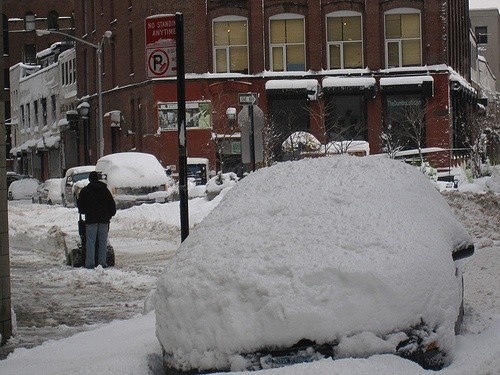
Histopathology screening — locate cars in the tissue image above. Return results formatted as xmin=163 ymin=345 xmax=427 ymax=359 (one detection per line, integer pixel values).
xmin=8 ymin=177 xmax=39 ymax=200
xmin=157 ymin=155 xmax=474 ymax=375
xmin=32 ymin=177 xmax=65 ymax=204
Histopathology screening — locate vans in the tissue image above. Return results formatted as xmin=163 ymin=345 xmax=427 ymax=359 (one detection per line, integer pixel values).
xmin=61 ymin=164 xmax=100 ymax=208
xmin=95 ymin=152 xmax=168 ymax=207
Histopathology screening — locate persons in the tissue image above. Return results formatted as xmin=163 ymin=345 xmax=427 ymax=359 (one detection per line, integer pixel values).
xmin=76 ymin=171 xmax=116 ymax=269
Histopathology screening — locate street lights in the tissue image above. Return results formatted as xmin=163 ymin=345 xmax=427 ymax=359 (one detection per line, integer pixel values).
xmin=36 ymin=29 xmax=113 ymax=161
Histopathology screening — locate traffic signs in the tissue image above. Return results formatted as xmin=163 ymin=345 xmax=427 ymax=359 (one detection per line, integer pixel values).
xmin=238 ymin=92 xmax=258 ymax=106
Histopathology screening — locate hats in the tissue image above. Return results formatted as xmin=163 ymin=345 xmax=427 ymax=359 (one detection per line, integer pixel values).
xmin=89 ymin=171 xmax=98 ymax=181
